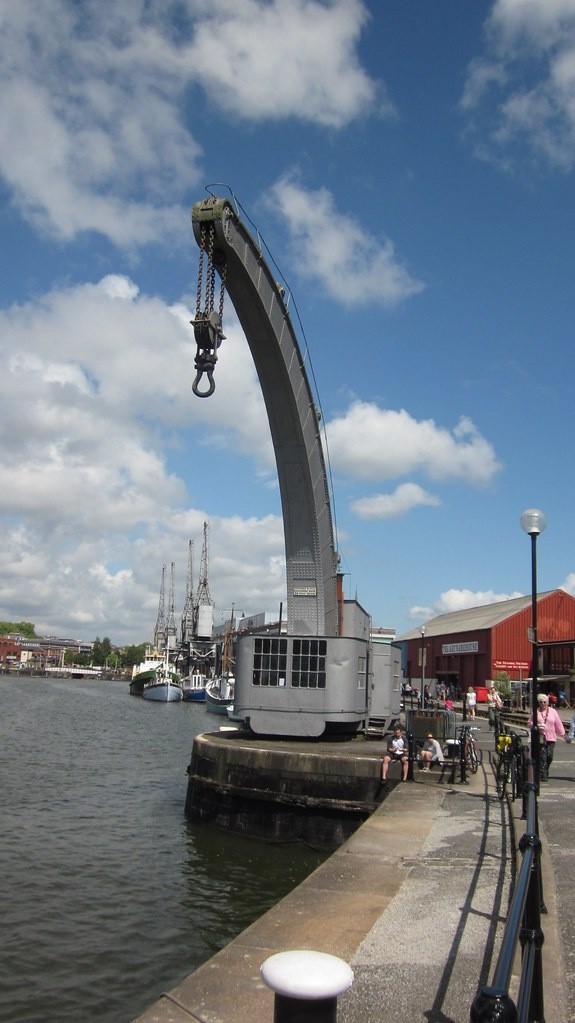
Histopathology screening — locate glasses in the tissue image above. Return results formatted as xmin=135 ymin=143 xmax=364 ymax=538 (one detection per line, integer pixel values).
xmin=427 ymin=737 xmax=433 ymax=739
xmin=540 ymin=701 xmax=546 ymax=704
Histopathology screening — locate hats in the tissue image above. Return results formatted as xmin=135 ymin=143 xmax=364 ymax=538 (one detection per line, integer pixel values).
xmin=424 ymin=684 xmax=429 ymax=689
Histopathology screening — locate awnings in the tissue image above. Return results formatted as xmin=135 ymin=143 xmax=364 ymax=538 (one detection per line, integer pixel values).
xmin=523 ymin=675 xmax=570 ymax=682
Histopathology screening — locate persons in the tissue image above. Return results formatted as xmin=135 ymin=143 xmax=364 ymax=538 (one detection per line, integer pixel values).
xmin=466 ymin=686 xmax=476 ymax=721
xmin=382 ymin=728 xmax=409 ymax=782
xmin=559 ymin=689 xmax=565 ymax=707
xmin=399 ymin=681 xmax=462 ymax=717
xmin=548 ymin=692 xmax=558 ymax=708
xmin=487 ymin=687 xmax=503 ymax=734
xmin=420 ymin=732 xmax=444 ymax=770
xmin=527 ymin=693 xmax=575 ymax=782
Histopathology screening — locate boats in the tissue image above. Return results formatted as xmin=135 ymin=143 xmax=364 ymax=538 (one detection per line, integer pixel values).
xmin=181 ymin=666 xmax=211 ymax=702
xmin=143 ymin=637 xmax=184 ymax=702
xmin=226 ymin=706 xmax=246 ymax=723
xmin=129 ymin=645 xmax=181 ymax=697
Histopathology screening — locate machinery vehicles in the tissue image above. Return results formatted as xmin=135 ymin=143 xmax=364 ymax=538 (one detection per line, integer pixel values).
xmin=175 ymin=175 xmax=414 ymax=741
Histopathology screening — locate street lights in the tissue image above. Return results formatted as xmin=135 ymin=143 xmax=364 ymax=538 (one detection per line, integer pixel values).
xmin=419 ymin=626 xmax=428 ymax=711
xmin=520 ymin=508 xmax=549 ymax=798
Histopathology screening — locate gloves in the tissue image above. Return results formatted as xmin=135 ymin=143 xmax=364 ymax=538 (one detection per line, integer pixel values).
xmin=564 ymin=734 xmax=571 ymax=743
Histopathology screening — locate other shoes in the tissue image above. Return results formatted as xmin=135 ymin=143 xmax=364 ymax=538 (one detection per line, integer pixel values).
xmin=422 ymin=767 xmax=426 ymax=770
xmin=489 ymin=725 xmax=492 ymax=733
xmin=542 ymin=773 xmax=548 ymax=781
xmin=381 ymin=779 xmax=387 ymax=784
xmin=402 ymin=778 xmax=406 ymax=782
xmin=423 ymin=768 xmax=430 ymax=773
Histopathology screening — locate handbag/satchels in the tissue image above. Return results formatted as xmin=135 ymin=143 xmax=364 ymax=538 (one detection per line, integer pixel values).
xmin=497 ymin=702 xmax=504 ymax=710
xmin=540 ymin=733 xmax=547 ymax=747
xmin=538 ymin=724 xmax=545 ymax=730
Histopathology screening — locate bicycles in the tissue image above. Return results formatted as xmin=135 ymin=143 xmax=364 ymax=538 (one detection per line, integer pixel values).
xmin=491 ymin=728 xmax=530 ymax=802
xmin=455 ymin=724 xmax=482 ymax=773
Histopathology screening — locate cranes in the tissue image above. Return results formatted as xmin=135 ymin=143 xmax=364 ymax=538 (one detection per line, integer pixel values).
xmin=148 ymin=519 xmax=216 ymax=652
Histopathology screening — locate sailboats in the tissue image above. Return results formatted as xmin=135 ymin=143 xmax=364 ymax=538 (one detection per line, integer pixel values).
xmin=204 ymin=602 xmax=243 ymax=716
